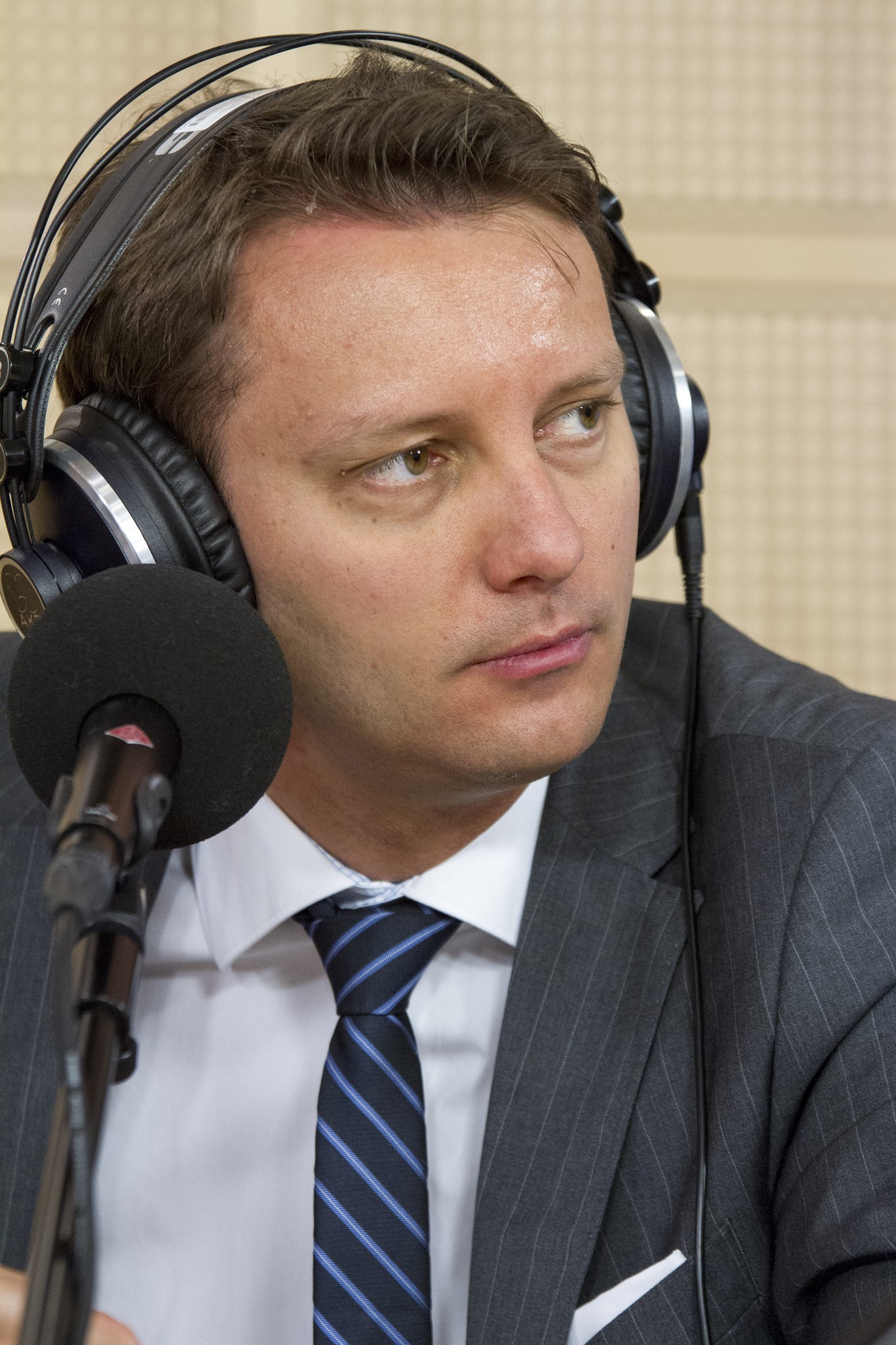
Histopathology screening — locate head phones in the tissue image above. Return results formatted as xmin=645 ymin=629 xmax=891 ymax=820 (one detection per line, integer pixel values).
xmin=1 ymin=28 xmax=709 ymax=638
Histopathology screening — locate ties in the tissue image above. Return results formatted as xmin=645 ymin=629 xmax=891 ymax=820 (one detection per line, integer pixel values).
xmin=291 ymin=894 xmax=462 ymax=1345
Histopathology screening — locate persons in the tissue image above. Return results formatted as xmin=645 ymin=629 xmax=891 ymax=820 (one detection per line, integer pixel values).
xmin=0 ymin=56 xmax=896 ymax=1345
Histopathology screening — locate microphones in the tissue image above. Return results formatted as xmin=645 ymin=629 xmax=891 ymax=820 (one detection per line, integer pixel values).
xmin=8 ymin=563 xmax=293 ymax=934
